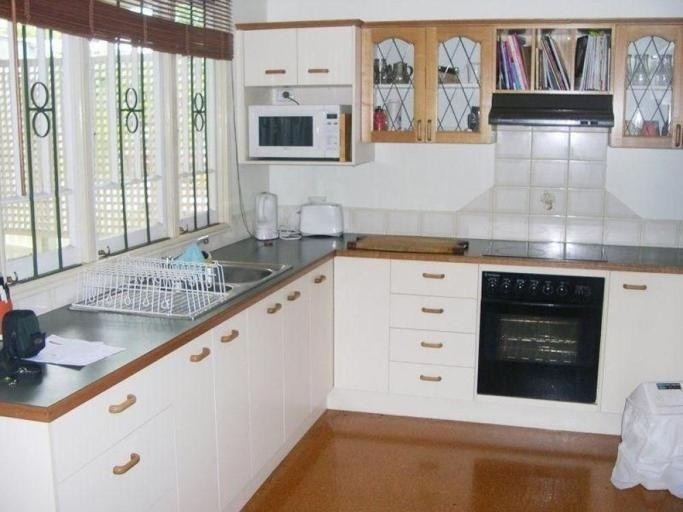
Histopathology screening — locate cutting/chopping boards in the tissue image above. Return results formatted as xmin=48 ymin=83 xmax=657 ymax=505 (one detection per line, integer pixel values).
xmin=347 ymin=234 xmax=469 ymax=254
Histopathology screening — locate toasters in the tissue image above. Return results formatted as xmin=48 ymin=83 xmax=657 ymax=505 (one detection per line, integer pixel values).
xmin=296 ymin=203 xmax=344 ymax=238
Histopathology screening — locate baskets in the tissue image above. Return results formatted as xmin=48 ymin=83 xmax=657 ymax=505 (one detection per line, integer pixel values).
xmin=69 ymin=255 xmax=229 ymax=320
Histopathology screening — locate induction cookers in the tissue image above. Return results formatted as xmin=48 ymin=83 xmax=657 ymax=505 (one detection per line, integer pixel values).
xmin=481 ymin=240 xmax=609 ymax=262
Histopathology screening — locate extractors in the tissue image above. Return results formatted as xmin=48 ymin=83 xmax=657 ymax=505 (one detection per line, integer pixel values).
xmin=488 ymin=93 xmax=614 ymax=127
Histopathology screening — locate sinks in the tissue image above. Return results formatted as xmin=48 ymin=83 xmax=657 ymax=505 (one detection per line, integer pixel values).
xmin=212 ymin=265 xmax=273 ymax=285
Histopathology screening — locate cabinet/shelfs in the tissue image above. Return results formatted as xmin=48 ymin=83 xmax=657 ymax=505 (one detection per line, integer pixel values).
xmin=248 ymin=274 xmax=310 ymax=498
xmin=327 ymin=255 xmax=479 ymax=421
xmin=599 ymin=271 xmax=683 ymax=437
xmin=308 ymin=259 xmax=334 ymax=427
xmin=0 ymin=348 xmax=176 ymax=512
xmin=495 ymin=19 xmax=615 ymax=94
xmin=234 ymin=18 xmax=374 ymax=168
xmin=175 ymin=310 xmax=252 ymax=512
xmin=608 ymin=17 xmax=683 ymax=149
xmin=361 ymin=19 xmax=497 ymax=144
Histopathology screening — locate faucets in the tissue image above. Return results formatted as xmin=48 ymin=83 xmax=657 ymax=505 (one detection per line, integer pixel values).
xmin=158 ymin=234 xmax=210 ymax=270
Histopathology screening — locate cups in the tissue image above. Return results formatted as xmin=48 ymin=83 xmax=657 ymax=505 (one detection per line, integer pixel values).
xmin=374 ymin=59 xmax=387 ymax=83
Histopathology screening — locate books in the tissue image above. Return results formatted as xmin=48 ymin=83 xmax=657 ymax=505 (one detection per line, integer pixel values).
xmin=496 ymin=27 xmax=613 ymax=91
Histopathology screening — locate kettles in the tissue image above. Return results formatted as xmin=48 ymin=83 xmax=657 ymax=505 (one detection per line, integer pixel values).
xmin=254 ymin=192 xmax=279 ymax=240
xmin=385 ymin=98 xmax=401 ymax=132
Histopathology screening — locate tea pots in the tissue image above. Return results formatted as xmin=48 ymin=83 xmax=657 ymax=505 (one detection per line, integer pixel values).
xmin=388 ymin=61 xmax=413 ymax=84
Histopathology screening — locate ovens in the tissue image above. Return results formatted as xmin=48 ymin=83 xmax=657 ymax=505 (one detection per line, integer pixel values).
xmin=477 ymin=271 xmax=605 ymax=403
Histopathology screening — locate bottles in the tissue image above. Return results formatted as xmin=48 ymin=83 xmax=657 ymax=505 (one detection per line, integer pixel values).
xmin=633 ymin=55 xmax=649 ymax=84
xmin=374 ymin=106 xmax=386 ymax=130
xmin=661 ymin=54 xmax=672 ymax=84
xmin=649 ymin=56 xmax=661 ymax=84
xmin=626 ymin=54 xmax=632 ymax=84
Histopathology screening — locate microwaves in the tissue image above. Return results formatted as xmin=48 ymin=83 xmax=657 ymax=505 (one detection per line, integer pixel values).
xmin=248 ymin=104 xmax=351 ymax=161
